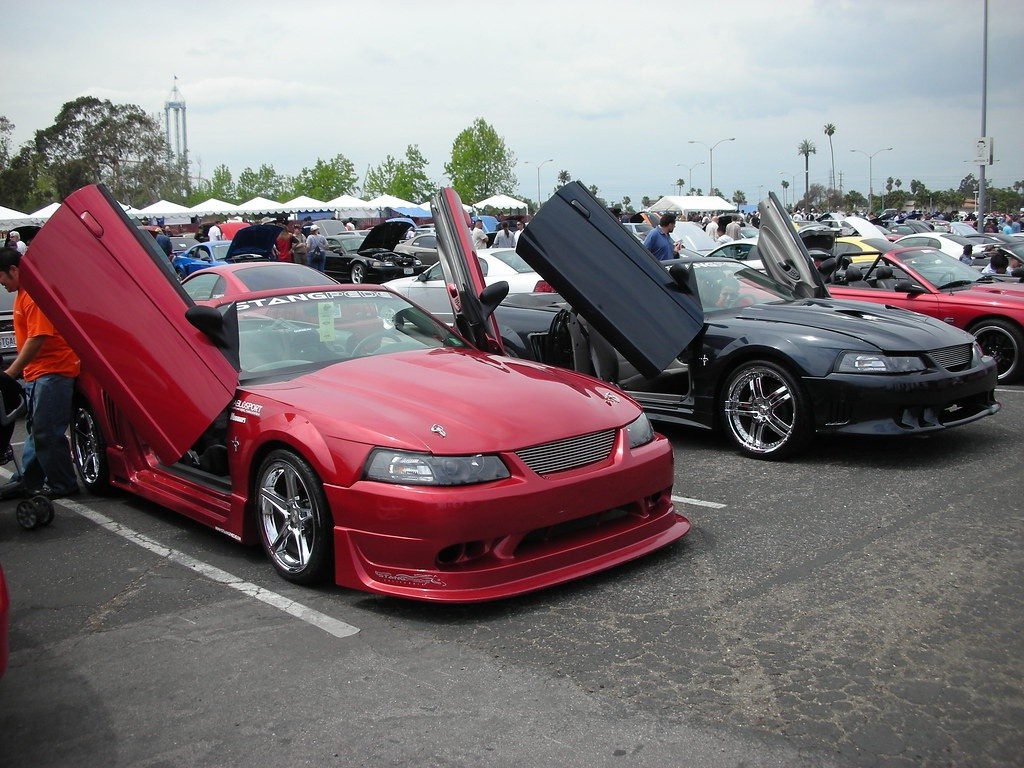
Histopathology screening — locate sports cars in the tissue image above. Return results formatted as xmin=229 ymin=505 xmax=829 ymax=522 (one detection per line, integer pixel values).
xmin=828 ymin=243 xmax=1024 ymax=381
xmin=455 ymin=253 xmax=1001 ymax=457
xmin=18 ymin=185 xmax=699 ymax=608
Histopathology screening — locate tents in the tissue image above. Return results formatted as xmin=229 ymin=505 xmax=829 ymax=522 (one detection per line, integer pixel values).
xmin=648 ymin=195 xmax=737 ymax=220
xmin=0 ymin=201 xmax=64 ymax=232
xmin=115 ymin=193 xmax=529 ymax=220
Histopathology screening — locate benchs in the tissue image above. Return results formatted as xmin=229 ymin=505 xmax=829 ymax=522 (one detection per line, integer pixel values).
xmin=240 ymin=317 xmax=336 ymax=369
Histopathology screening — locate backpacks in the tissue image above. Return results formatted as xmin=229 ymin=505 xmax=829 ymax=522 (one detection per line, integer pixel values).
xmin=311 ymin=234 xmax=322 ymax=261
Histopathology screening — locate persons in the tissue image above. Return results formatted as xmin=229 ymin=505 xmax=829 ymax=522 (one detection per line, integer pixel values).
xmin=471 ymin=219 xmax=526 ymax=249
xmin=789 ymin=207 xmax=833 ymax=224
xmin=714 ymin=277 xmax=739 ymax=308
xmin=959 ymin=243 xmax=1022 ymax=275
xmin=644 ymin=212 xmax=680 ymax=260
xmin=621 ymin=212 xmax=629 ymax=223
xmin=261 ymin=217 xmax=329 ymax=272
xmin=0 ymin=231 xmax=26 ymax=255
xmin=894 ymin=210 xmax=977 ymax=223
xmin=191 ymin=216 xmax=227 ymax=243
xmin=982 ymin=211 xmax=1024 ymax=234
xmin=142 ymin=216 xmax=173 ymax=256
xmin=675 ymin=210 xmax=760 ymax=256
xmin=331 ymin=215 xmax=358 ymax=231
xmin=0 ymin=247 xmax=80 ymax=498
xmin=845 ymin=210 xmax=881 ymax=220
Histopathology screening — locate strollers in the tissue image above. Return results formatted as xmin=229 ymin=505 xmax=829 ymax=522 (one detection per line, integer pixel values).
xmin=0 ymin=360 xmax=55 ymax=532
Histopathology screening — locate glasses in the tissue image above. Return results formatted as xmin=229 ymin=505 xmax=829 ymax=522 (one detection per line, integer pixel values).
xmin=516 ymin=224 xmax=522 ymax=226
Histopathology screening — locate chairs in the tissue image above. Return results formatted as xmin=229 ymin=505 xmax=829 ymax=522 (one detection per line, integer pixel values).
xmin=571 ymin=310 xmax=689 ymax=387
xmin=875 ymin=266 xmax=899 ymax=289
xmin=845 ymin=267 xmax=873 ymax=288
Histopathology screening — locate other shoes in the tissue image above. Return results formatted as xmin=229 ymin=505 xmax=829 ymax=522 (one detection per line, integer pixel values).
xmin=33 ymin=484 xmax=81 ymax=500
xmin=0 ymin=480 xmax=33 ymax=496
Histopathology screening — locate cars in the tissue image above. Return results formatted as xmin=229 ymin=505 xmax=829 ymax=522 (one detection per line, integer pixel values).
xmin=1 ymin=211 xmax=1024 ymax=323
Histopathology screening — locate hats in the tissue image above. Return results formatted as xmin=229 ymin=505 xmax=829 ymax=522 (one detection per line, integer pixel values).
xmin=474 ymin=219 xmax=482 ymax=224
xmin=310 ymin=225 xmax=320 ymax=231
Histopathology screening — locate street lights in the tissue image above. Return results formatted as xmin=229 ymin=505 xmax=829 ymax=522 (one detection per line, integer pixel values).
xmin=675 ymin=161 xmax=705 ymax=196
xmin=780 ymin=170 xmax=809 ymax=204
xmin=850 ymin=147 xmax=893 ymax=215
xmin=688 ymin=136 xmax=736 ymax=194
xmin=524 ymin=158 xmax=554 ymax=209
